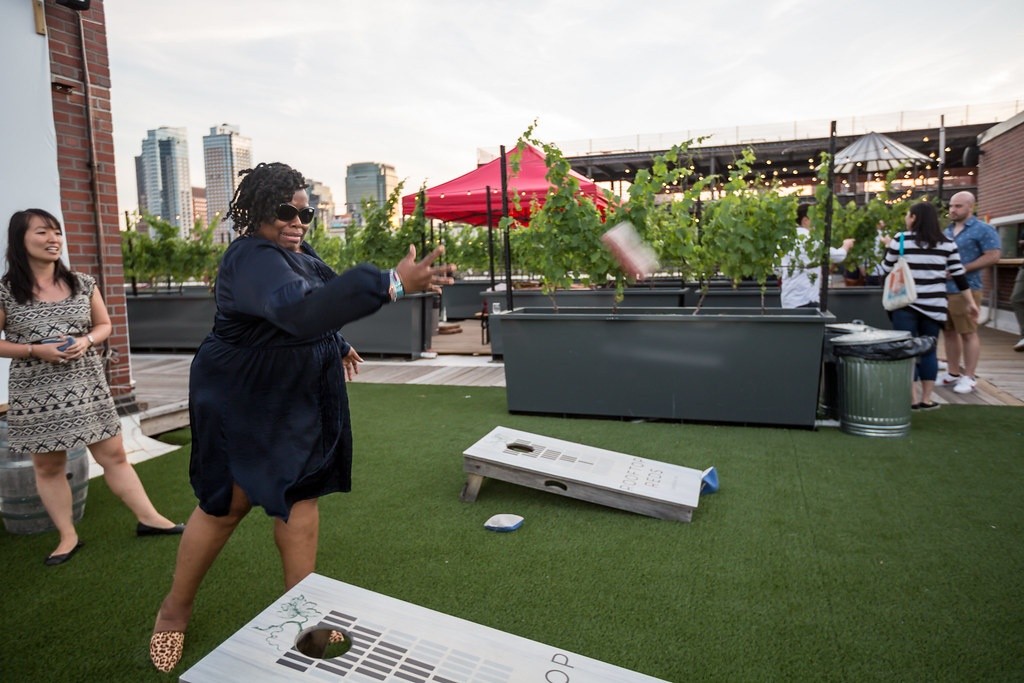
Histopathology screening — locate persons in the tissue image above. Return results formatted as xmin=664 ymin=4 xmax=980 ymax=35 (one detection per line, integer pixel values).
xmin=772 ymin=204 xmax=855 ymax=308
xmin=940 ymin=191 xmax=1002 ymax=394
xmin=1010 ymin=231 xmax=1024 ymax=352
xmin=0 ymin=209 xmax=186 ymax=564
xmin=151 ymin=162 xmax=456 ymax=677
xmin=873 ymin=203 xmax=979 ymax=413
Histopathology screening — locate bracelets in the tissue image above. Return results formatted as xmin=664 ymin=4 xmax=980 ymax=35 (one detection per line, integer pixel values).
xmin=389 ymin=266 xmax=406 ymax=303
xmin=28 ymin=343 xmax=33 ymax=358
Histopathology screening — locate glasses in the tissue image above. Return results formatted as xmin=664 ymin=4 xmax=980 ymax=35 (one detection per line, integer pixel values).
xmin=277 ymin=203 xmax=315 ymax=224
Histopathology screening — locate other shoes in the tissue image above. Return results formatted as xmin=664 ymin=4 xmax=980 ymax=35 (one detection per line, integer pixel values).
xmin=136 ymin=521 xmax=188 ymax=536
xmin=912 ymin=403 xmax=920 ymax=412
xmin=329 ymin=629 xmax=345 ymax=645
xmin=1013 ymin=338 xmax=1024 ymax=352
xmin=919 ymin=400 xmax=941 ymax=411
xmin=46 ymin=538 xmax=82 ymax=566
xmin=150 ymin=630 xmax=185 ymax=673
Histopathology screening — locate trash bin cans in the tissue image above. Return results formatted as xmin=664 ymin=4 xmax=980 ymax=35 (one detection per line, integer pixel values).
xmin=832 ymin=327 xmax=937 ymax=439
xmin=822 ymin=323 xmax=881 ymax=412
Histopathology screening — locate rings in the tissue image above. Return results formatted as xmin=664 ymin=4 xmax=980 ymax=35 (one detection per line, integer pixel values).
xmin=59 ymin=359 xmax=64 ymax=363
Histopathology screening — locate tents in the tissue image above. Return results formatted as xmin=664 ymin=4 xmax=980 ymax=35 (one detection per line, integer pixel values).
xmin=404 ymin=139 xmax=630 ymax=279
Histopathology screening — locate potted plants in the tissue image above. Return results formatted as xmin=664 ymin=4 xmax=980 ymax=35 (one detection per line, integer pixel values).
xmin=480 ymin=114 xmax=953 ymax=430
xmin=119 ymin=178 xmax=480 ymax=361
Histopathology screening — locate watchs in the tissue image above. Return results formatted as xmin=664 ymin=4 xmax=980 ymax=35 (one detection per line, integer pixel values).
xmin=85 ymin=334 xmax=94 ymax=349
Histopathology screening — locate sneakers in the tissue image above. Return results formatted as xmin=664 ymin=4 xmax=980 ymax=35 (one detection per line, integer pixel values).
xmin=933 ymin=372 xmax=962 ymax=387
xmin=952 ymin=376 xmax=978 ymax=395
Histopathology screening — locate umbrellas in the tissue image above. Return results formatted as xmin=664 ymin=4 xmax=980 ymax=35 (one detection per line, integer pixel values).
xmin=815 ymin=134 xmax=933 ymax=205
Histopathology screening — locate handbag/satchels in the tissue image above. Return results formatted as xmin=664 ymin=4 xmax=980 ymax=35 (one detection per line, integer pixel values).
xmin=882 ymin=232 xmax=918 ymax=311
